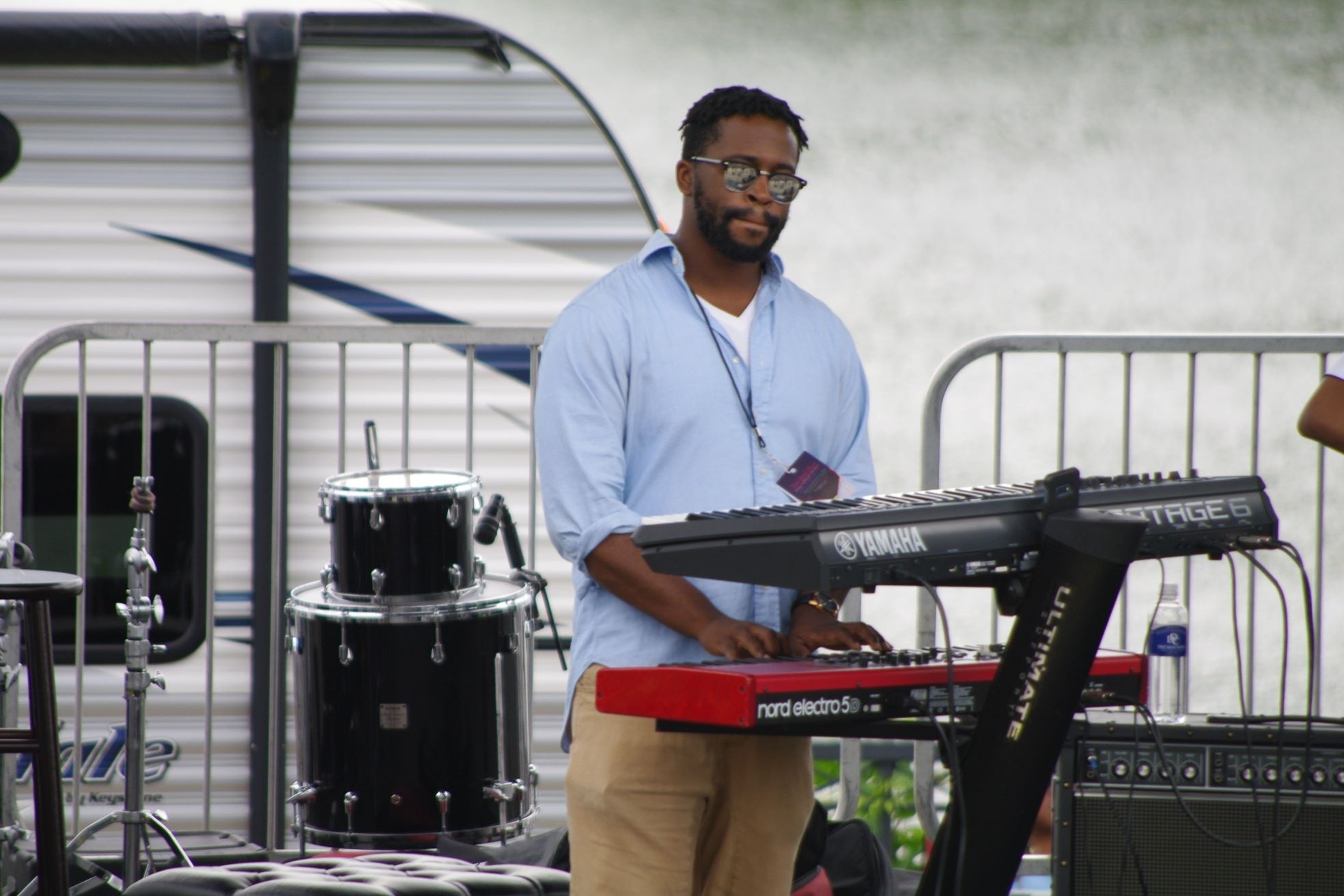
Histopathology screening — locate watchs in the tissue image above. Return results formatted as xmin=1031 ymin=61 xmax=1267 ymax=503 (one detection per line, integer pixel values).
xmin=791 ymin=592 xmax=840 ymax=620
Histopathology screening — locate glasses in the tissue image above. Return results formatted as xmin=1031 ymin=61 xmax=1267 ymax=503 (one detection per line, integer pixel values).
xmin=690 ymin=155 xmax=809 ymax=204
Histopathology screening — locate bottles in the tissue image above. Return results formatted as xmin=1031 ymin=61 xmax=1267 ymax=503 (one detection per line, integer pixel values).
xmin=1147 ymin=582 xmax=1189 ymax=723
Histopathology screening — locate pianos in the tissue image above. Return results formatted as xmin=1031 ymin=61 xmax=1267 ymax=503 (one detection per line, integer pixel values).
xmin=629 ymin=469 xmax=1281 ymax=894
xmin=593 ymin=646 xmax=1145 ymax=896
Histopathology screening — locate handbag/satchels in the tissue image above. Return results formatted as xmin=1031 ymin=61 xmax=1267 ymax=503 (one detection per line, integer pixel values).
xmin=546 ymin=800 xmax=894 ymax=895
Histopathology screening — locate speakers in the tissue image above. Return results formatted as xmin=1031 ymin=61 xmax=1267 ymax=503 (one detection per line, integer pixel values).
xmin=1057 ymin=721 xmax=1342 ymax=896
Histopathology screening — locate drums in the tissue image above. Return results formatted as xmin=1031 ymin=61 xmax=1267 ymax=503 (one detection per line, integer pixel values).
xmin=283 ymin=575 xmax=538 ymax=846
xmin=319 ymin=470 xmax=487 ymax=601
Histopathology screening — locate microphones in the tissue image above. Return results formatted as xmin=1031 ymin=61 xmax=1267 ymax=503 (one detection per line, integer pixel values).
xmin=471 ymin=494 xmax=505 ymax=545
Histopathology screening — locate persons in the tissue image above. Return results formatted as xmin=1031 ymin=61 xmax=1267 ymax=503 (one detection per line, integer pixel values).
xmin=1297 ymin=349 xmax=1344 ymax=453
xmin=535 ymin=87 xmax=895 ymax=896
xmin=1025 ymin=784 xmax=1051 ymax=855
